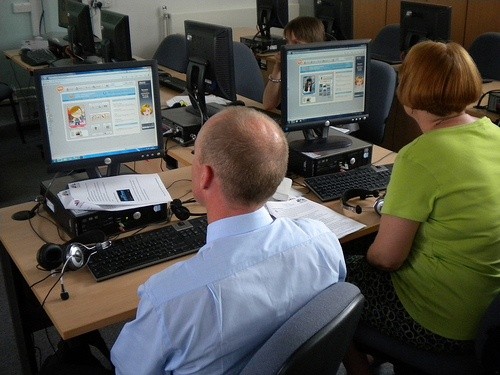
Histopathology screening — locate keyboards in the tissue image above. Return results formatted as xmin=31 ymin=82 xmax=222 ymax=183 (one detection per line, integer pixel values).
xmin=371 ymin=53 xmax=404 ymax=65
xmin=301 ymin=162 xmax=395 ymax=202
xmin=22 ymin=48 xmax=56 ymax=65
xmin=80 ymin=214 xmax=208 ymax=283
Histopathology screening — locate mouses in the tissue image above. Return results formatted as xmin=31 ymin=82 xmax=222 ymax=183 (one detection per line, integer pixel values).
xmin=19 ymin=48 xmax=31 ymax=56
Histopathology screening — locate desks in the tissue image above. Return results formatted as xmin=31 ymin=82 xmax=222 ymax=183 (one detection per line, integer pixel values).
xmin=0 ymin=25 xmax=500 ymax=375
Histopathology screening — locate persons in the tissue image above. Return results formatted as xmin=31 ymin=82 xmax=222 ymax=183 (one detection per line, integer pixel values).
xmin=108 ymin=106 xmax=347 ymax=375
xmin=262 ymin=16 xmax=324 ymax=110
xmin=341 ymin=39 xmax=500 ymax=375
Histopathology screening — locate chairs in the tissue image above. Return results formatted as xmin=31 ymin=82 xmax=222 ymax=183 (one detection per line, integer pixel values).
xmin=154 ymin=33 xmax=188 ymax=74
xmin=342 ymin=59 xmax=396 ymax=142
xmin=468 ymin=33 xmax=500 ymax=82
xmin=225 ymin=282 xmax=365 ymax=375
xmin=0 ymin=82 xmax=26 ymax=144
xmin=370 ymin=24 xmax=400 ymax=63
xmin=233 ymin=42 xmax=266 ymax=104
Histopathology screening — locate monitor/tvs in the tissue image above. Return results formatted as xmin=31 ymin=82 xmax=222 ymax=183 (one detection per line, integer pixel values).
xmin=33 ymin=59 xmax=165 ymax=180
xmin=256 ymin=0 xmax=290 ymax=40
xmin=398 ymin=0 xmax=452 ymax=58
xmin=99 ymin=9 xmax=133 ymax=62
xmin=313 ymin=0 xmax=355 ymax=41
xmin=57 ymin=0 xmax=97 ymax=65
xmin=278 ymin=38 xmax=372 ymax=152
xmin=183 ymin=19 xmax=239 ymax=119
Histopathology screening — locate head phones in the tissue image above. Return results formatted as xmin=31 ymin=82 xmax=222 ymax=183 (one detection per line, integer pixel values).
xmin=171 ymin=198 xmax=208 ymax=220
xmin=35 ymin=229 xmax=111 ymax=270
xmin=340 ymin=188 xmax=386 ymax=216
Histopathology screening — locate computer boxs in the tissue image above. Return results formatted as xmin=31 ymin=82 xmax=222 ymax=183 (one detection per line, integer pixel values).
xmin=159 ymin=76 xmax=186 ymax=92
xmin=45 ymin=33 xmax=104 ymax=57
xmin=40 ymin=162 xmax=171 ymax=244
xmin=240 ymin=34 xmax=284 ymax=55
xmin=160 ymin=101 xmax=230 ymax=147
xmin=283 ymin=125 xmax=373 ymax=180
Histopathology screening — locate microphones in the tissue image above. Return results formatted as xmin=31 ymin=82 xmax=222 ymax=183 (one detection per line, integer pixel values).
xmin=59 ymin=254 xmax=79 ymax=301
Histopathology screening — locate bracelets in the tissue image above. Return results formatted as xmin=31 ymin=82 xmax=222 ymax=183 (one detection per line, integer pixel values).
xmin=268 ymin=75 xmax=281 ymax=83
